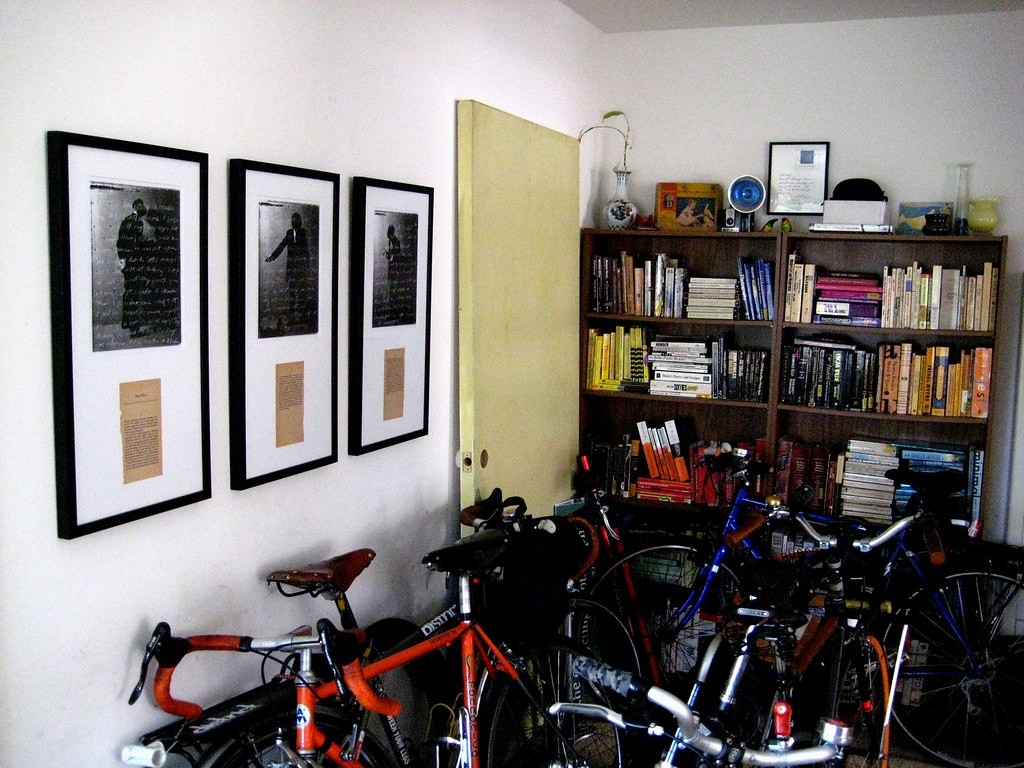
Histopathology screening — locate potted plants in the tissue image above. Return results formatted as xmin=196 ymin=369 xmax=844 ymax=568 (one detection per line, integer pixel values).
xmin=578 ymin=110 xmax=638 ymax=231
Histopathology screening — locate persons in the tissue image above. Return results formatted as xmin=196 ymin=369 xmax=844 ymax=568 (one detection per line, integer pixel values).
xmin=265 ymin=213 xmax=313 ymax=324
xmin=678 ymin=198 xmax=714 ymax=227
xmin=384 ymin=225 xmax=405 ymax=309
xmin=116 ymin=199 xmax=167 ymax=337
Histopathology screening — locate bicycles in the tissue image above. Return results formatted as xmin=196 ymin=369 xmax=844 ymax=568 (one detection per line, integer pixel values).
xmin=128 ymin=442 xmax=1024 ymax=768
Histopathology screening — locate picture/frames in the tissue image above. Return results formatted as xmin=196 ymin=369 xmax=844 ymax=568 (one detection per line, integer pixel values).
xmin=347 ymin=178 xmax=434 ymax=456
xmin=766 ymin=142 xmax=830 ymax=215
xmin=46 ymin=131 xmax=212 ymax=539
xmin=227 ymin=158 xmax=340 ymax=490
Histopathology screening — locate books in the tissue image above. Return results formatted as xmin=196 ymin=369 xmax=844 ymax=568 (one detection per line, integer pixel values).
xmin=588 ymin=246 xmax=997 ymax=536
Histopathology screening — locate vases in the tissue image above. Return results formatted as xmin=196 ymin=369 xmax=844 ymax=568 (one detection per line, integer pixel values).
xmin=966 ymin=196 xmax=1002 ymax=239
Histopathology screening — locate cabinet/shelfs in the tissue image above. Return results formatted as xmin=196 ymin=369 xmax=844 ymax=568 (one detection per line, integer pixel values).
xmin=577 ymin=227 xmax=1010 ymax=540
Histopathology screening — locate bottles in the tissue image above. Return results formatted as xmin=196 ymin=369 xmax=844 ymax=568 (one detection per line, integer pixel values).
xmin=951 ymin=164 xmax=974 ymax=236
